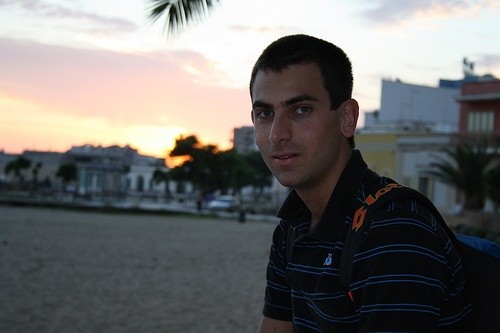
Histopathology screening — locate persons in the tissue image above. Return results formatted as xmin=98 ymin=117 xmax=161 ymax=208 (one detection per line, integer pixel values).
xmin=250 ymin=34 xmax=473 ymax=333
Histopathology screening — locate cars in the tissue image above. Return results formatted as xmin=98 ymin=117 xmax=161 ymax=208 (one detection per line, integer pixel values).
xmin=209 ymin=195 xmax=241 ymax=213
